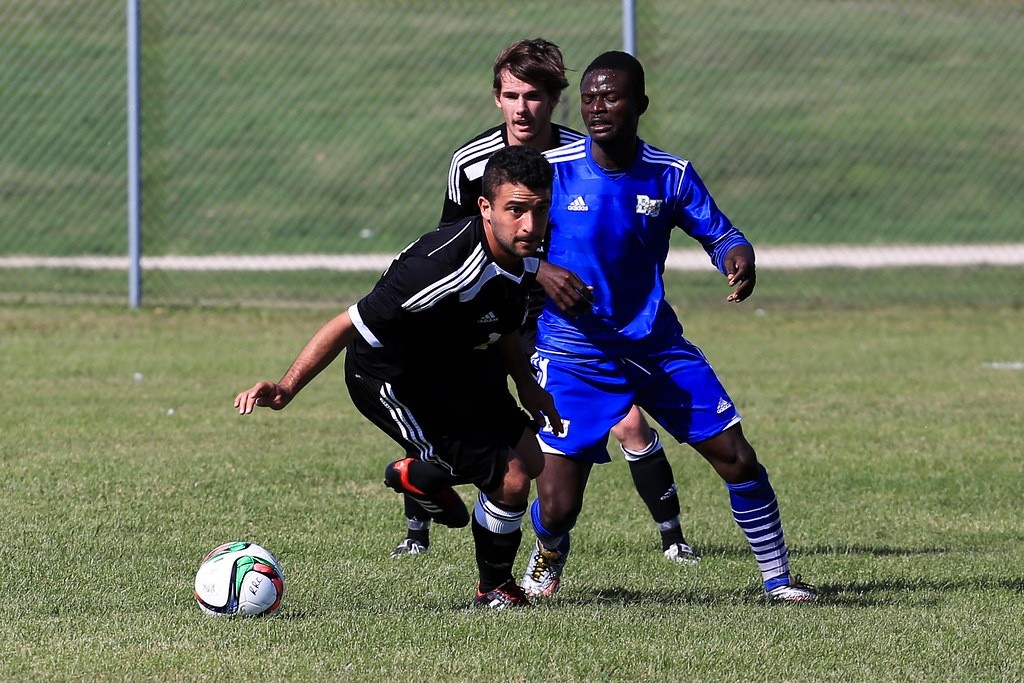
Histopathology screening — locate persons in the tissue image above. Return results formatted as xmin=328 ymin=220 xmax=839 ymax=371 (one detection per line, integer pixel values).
xmin=506 ymin=49 xmax=830 ymax=605
xmin=384 ymin=36 xmax=703 ymax=561
xmin=234 ymin=144 xmax=564 ymax=612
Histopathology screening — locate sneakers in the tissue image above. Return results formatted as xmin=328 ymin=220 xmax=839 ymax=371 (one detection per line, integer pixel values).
xmin=662 ymin=544 xmax=702 ymax=565
xmin=521 ymin=533 xmax=570 ymax=597
xmin=474 ymin=579 xmax=534 ymax=611
xmin=765 ymin=574 xmax=821 ymax=603
xmin=389 ymin=537 xmax=428 ymax=559
xmin=384 ymin=458 xmax=469 ymax=528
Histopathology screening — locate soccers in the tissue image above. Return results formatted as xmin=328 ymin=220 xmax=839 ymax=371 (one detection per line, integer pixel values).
xmin=194 ymin=541 xmax=284 ymax=619
